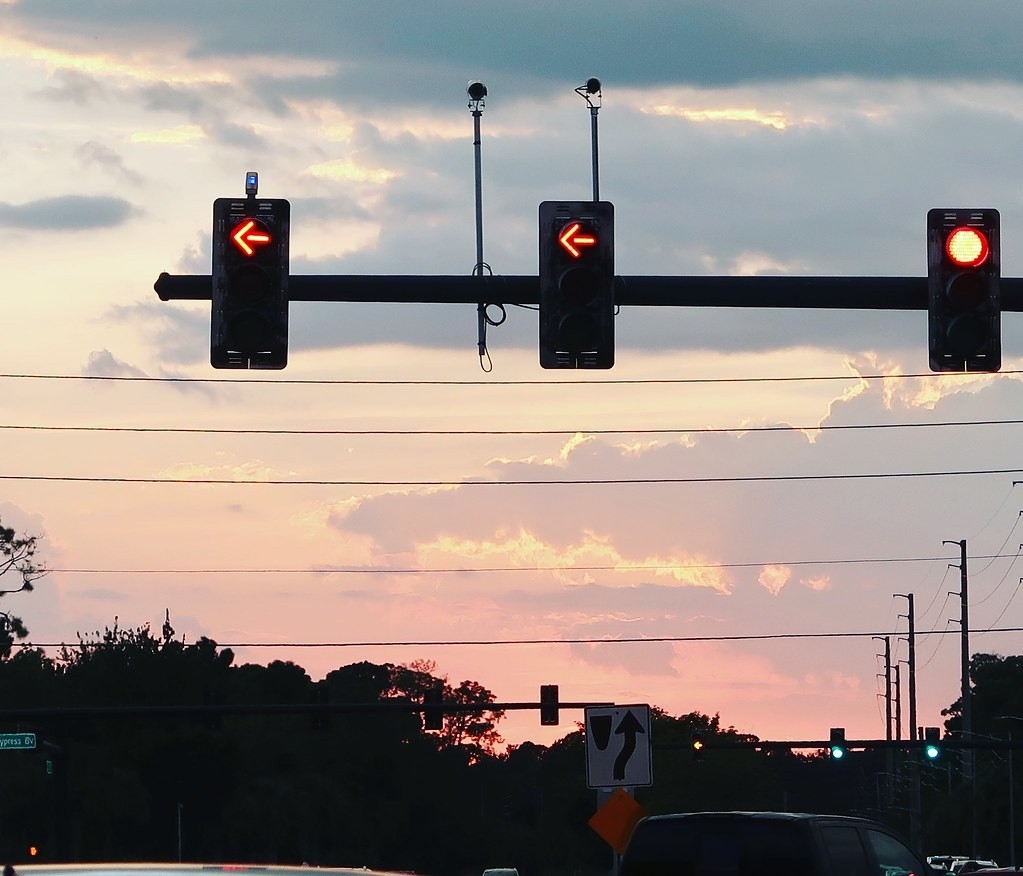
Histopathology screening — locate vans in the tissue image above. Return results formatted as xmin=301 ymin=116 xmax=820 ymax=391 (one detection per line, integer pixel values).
xmin=617 ymin=808 xmax=936 ymax=876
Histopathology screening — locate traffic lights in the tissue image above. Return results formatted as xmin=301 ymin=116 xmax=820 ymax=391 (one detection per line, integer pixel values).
xmin=926 ymin=727 xmax=941 ymax=760
xmin=535 ymin=199 xmax=617 ymax=371
xmin=690 ymin=732 xmax=706 ymax=760
xmin=829 ymin=728 xmax=845 ymax=765
xmin=209 ymin=197 xmax=291 ymax=372
xmin=923 ymin=207 xmax=1004 ymax=373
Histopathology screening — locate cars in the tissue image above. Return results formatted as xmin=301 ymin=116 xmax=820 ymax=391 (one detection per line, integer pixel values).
xmin=880 ymin=855 xmax=1023 ymax=876
xmin=481 ymin=866 xmax=519 ymax=876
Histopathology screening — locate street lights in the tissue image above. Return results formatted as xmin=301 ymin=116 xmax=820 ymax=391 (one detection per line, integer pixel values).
xmin=901 ymin=758 xmax=952 ymax=799
xmin=949 ymin=714 xmax=1023 ymax=866
